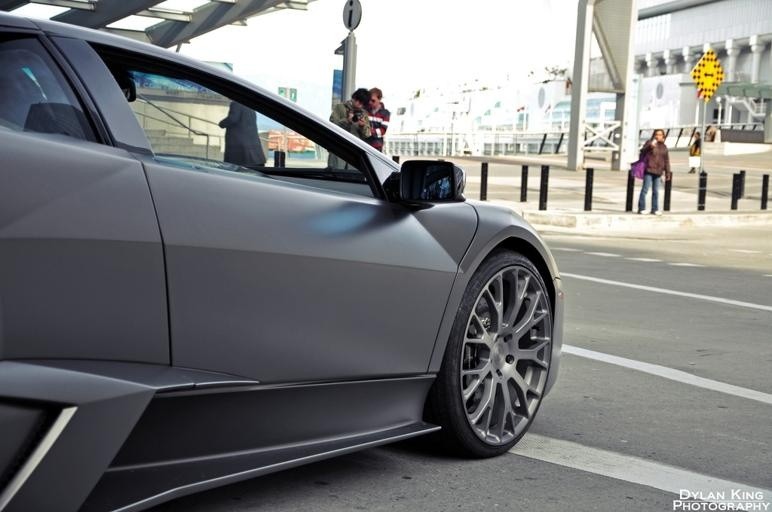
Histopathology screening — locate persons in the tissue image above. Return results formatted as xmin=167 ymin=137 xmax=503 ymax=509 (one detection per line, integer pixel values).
xmin=362 ymin=88 xmax=391 ymax=155
xmin=635 ymin=129 xmax=671 ymax=216
xmin=216 ymin=98 xmax=267 ymax=169
xmin=687 ymin=132 xmax=701 ymax=173
xmin=325 ymin=87 xmax=374 ymax=174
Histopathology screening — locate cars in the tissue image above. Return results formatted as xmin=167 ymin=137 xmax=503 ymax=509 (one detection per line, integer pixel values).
xmin=0 ymin=11 xmax=564 ymax=512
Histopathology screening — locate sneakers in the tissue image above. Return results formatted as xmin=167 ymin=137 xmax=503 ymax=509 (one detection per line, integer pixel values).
xmin=639 ymin=209 xmax=663 ymax=215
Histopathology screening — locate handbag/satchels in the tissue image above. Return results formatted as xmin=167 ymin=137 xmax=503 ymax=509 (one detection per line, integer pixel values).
xmin=628 ymin=154 xmax=649 ymax=178
xmin=688 ymin=154 xmax=700 ymax=168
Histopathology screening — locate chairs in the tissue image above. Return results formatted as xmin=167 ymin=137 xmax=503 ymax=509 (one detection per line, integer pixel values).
xmin=0 ymin=57 xmax=59 ymax=133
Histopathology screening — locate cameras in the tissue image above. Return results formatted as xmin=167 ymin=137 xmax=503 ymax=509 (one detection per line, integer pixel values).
xmin=350 ymin=106 xmax=364 ymax=125
